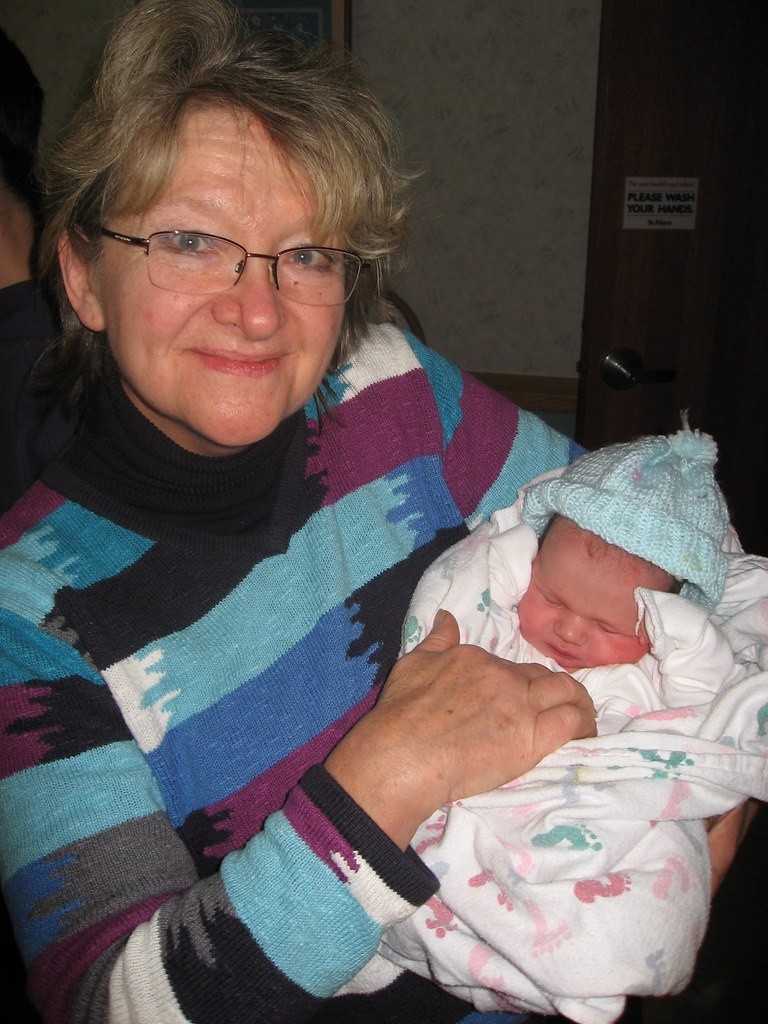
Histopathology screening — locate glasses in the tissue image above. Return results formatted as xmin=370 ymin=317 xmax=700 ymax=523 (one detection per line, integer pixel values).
xmin=103 ymin=228 xmax=371 ymax=307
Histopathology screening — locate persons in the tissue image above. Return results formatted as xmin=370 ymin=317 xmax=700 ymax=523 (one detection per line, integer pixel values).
xmin=0 ymin=0 xmax=759 ymax=1024
xmin=374 ymin=429 xmax=768 ymax=1024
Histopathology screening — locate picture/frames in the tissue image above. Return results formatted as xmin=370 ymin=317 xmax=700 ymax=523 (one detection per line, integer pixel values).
xmin=238 ymin=1 xmax=353 ymax=52
